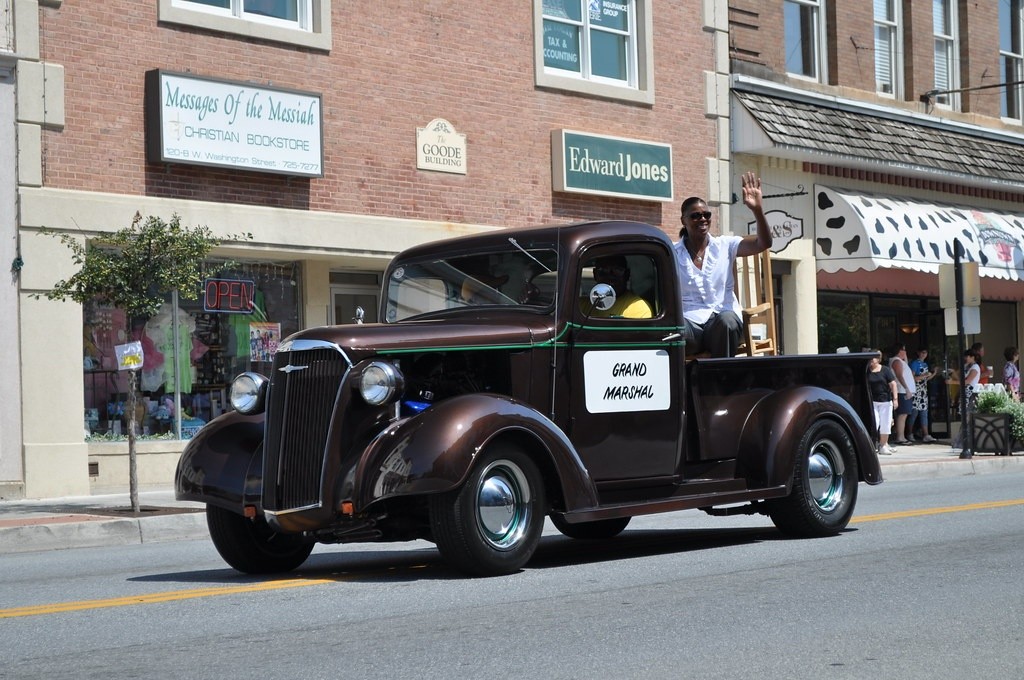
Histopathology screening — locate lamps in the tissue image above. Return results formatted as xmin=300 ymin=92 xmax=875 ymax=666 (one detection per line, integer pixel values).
xmin=899 ymin=313 xmax=919 ymax=335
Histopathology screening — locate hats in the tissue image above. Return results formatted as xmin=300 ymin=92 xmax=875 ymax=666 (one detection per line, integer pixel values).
xmin=836 ymin=346 xmax=850 ymax=354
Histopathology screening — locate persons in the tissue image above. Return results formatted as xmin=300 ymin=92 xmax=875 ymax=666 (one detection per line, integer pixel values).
xmin=906 ymin=346 xmax=939 ymax=444
xmin=865 ymin=347 xmax=899 ymax=454
xmin=250 ymin=329 xmax=275 ymax=361
xmin=946 ymin=349 xmax=981 ymax=449
xmin=459 ymin=272 xmax=508 ymax=305
xmin=580 ymin=254 xmax=654 ymax=319
xmin=672 ymin=171 xmax=772 ymax=359
xmin=886 ymin=343 xmax=917 ymax=445
xmin=971 ymin=343 xmax=990 ymax=384
xmin=1002 ymin=345 xmax=1020 ymax=402
xmin=150 ymin=302 xmax=196 ymax=422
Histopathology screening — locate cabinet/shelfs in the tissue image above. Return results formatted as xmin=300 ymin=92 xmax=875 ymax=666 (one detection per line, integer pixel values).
xmin=186 ymin=310 xmax=230 ymax=421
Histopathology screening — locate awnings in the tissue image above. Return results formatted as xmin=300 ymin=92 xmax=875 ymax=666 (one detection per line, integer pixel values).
xmin=815 ymin=182 xmax=1024 ymax=283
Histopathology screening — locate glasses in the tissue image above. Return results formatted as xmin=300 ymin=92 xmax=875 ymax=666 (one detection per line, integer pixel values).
xmin=683 ymin=212 xmax=712 ymax=220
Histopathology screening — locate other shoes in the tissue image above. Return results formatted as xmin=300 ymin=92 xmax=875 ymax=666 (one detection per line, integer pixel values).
xmin=886 ymin=445 xmax=897 ymax=452
xmin=895 ymin=437 xmax=912 ymax=445
xmin=907 ymin=433 xmax=915 ymax=442
xmin=923 ymin=435 xmax=937 ymax=442
xmin=879 ymin=445 xmax=892 ymax=455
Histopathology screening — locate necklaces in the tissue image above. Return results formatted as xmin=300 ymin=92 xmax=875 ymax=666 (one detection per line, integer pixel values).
xmin=686 ymin=242 xmax=708 ymax=261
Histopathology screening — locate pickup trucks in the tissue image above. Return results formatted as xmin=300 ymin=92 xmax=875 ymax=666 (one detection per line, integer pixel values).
xmin=174 ymin=219 xmax=882 ymax=576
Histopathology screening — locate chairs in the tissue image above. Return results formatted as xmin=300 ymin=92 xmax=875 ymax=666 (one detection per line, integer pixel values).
xmin=733 ymin=248 xmax=778 ymax=357
xmin=490 ymin=263 xmax=532 ymax=304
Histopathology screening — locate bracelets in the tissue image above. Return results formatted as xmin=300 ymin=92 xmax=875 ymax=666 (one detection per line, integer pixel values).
xmin=894 ymin=398 xmax=898 ymax=400
